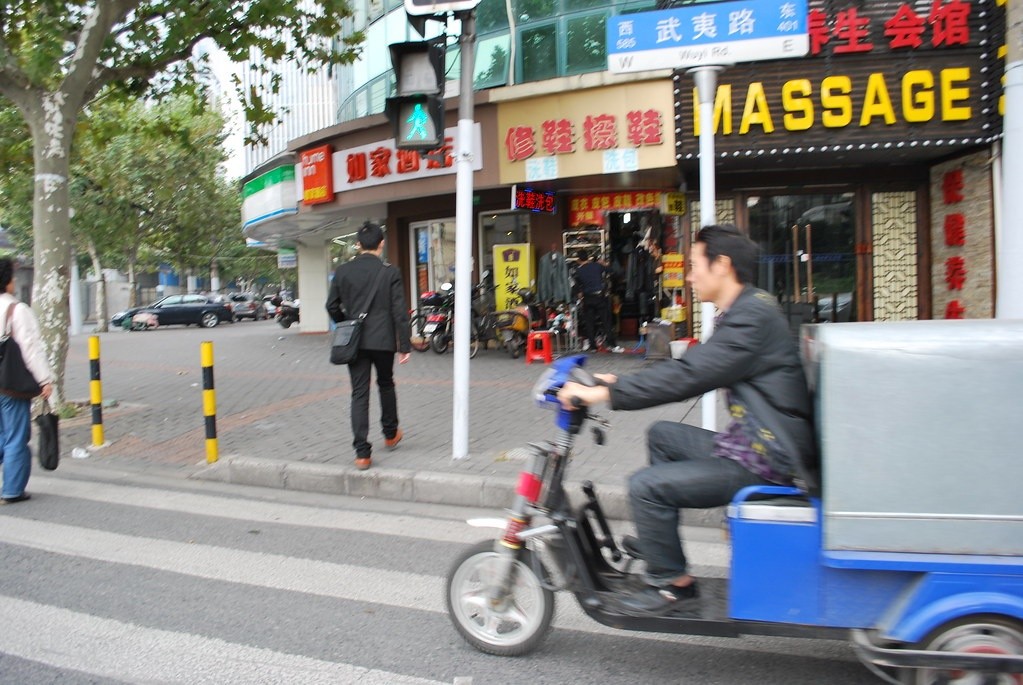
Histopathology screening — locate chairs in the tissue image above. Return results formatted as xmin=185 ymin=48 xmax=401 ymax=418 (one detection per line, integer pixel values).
xmin=725 ymin=485 xmax=821 ymax=521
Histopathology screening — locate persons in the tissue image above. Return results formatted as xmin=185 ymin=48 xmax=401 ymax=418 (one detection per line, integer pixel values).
xmin=572 ymin=249 xmax=625 ymax=354
xmin=0 ymin=256 xmax=54 ymax=504
xmin=557 ymin=223 xmax=821 ymax=619
xmin=325 ymin=223 xmax=411 ymax=471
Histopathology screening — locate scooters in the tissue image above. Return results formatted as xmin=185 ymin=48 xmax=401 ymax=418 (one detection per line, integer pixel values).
xmin=442 ymin=321 xmax=1023 ymax=685
xmin=496 ymin=278 xmax=543 ymax=358
xmin=271 ymin=297 xmax=299 ymax=328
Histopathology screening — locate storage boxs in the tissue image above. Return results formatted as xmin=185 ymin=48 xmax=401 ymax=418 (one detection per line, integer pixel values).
xmin=661 ymin=307 xmax=686 ymax=322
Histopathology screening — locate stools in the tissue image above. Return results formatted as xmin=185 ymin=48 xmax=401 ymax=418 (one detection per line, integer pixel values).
xmin=526 ymin=329 xmax=553 ymax=364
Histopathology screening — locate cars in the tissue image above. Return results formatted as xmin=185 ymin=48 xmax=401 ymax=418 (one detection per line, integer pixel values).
xmin=263 ymin=295 xmax=279 ymax=317
xmin=111 ymin=293 xmax=233 ymax=330
xmin=225 ymin=292 xmax=268 ymax=321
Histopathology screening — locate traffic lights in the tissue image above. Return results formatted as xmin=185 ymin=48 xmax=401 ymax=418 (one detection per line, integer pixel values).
xmin=385 ymin=43 xmax=446 ymax=151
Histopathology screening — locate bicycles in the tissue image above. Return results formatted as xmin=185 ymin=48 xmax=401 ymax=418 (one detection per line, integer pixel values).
xmin=406 ymin=270 xmax=497 ymax=359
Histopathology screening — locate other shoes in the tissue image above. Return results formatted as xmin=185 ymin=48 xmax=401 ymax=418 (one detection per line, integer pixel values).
xmin=383 ymin=431 xmax=402 ymax=448
xmin=355 ymin=457 xmax=373 ymax=471
xmin=622 ymin=576 xmax=703 ymax=611
xmin=622 ymin=532 xmax=646 ymax=561
xmin=1 ymin=492 xmax=32 ymax=505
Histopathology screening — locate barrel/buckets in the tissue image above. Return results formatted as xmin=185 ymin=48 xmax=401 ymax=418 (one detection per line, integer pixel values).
xmin=669 ymin=339 xmax=691 ymax=359
xmin=645 ymin=321 xmax=672 ymax=358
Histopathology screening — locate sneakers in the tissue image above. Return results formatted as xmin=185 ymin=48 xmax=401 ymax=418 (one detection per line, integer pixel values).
xmin=611 ymin=346 xmax=625 ymax=353
xmin=580 ymin=345 xmax=591 ymax=351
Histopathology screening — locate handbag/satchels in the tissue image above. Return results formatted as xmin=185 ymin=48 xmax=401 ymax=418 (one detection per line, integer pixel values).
xmin=0 ymin=302 xmax=41 ymax=399
xmin=329 ymin=319 xmax=365 ymax=366
xmin=34 ymin=400 xmax=59 ymax=471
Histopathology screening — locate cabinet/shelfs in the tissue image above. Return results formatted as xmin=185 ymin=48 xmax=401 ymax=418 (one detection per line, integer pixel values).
xmin=562 ymin=229 xmax=607 ymax=324
